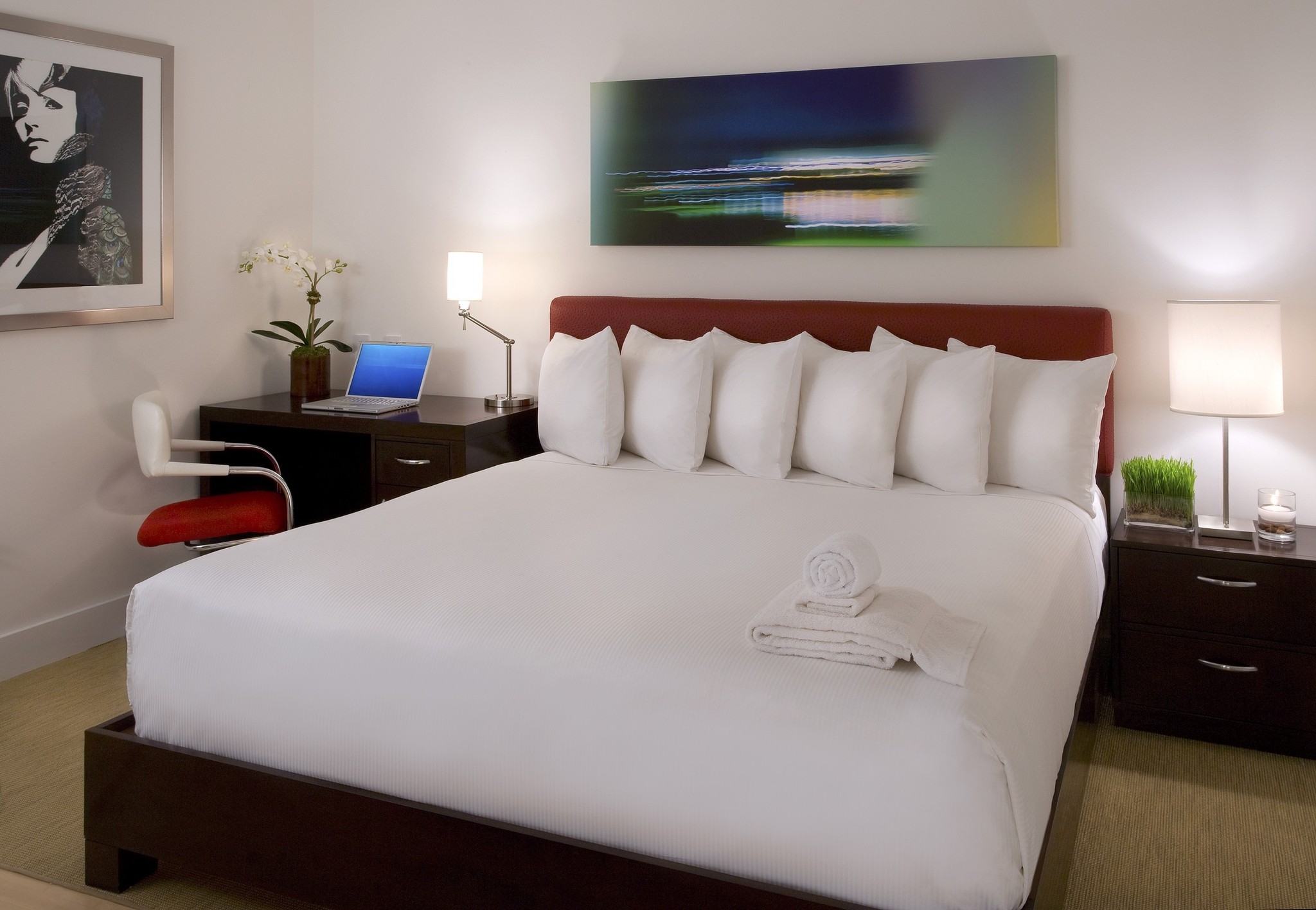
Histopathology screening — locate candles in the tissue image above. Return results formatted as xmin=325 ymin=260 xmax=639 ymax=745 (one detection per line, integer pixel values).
xmin=1261 ymin=490 xmax=1292 ymax=524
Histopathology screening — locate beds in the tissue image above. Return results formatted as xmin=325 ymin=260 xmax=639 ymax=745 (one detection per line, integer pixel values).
xmin=80 ymin=294 xmax=1128 ymax=910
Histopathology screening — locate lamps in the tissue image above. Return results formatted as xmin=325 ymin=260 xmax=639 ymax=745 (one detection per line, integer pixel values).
xmin=448 ymin=252 xmax=534 ymax=408
xmin=1165 ymin=298 xmax=1284 ymax=541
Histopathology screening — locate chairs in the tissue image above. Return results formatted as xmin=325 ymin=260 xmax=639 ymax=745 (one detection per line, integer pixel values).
xmin=133 ymin=392 xmax=293 ymax=559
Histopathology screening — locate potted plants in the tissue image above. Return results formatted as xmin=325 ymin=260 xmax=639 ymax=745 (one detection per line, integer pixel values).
xmin=1118 ymin=456 xmax=1197 ymax=535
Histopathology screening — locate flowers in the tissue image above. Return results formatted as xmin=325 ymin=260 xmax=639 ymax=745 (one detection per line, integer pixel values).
xmin=237 ymin=245 xmax=355 ymax=356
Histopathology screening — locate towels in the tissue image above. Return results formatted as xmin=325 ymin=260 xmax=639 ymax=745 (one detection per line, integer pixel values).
xmin=742 ymin=529 xmax=987 ymax=688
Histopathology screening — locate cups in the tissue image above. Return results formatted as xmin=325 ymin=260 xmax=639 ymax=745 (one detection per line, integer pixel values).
xmin=1256 ymin=487 xmax=1297 ymax=543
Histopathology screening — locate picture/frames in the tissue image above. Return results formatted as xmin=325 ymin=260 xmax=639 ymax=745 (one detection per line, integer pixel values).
xmin=1 ymin=11 xmax=181 ymax=336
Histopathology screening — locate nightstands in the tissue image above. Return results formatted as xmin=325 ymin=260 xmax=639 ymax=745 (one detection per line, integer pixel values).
xmin=1101 ymin=508 xmax=1316 ymax=759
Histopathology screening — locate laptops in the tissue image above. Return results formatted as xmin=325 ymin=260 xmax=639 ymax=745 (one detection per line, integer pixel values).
xmin=301 ymin=340 xmax=434 ymax=414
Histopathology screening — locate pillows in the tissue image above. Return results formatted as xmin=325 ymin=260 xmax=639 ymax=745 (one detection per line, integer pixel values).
xmin=700 ymin=328 xmax=801 ymax=481
xmin=785 ymin=329 xmax=906 ymax=491
xmin=869 ymin=324 xmax=994 ymax=497
xmin=944 ymin=338 xmax=1116 ymax=516
xmin=617 ymin=324 xmax=717 ymax=471
xmin=535 ymin=326 xmax=625 ymax=466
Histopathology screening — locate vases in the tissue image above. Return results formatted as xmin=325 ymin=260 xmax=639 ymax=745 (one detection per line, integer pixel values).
xmin=291 ymin=355 xmax=332 ymax=396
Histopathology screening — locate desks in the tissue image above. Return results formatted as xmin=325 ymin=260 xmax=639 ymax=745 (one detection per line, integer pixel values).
xmin=193 ymin=393 xmax=542 ymax=558
